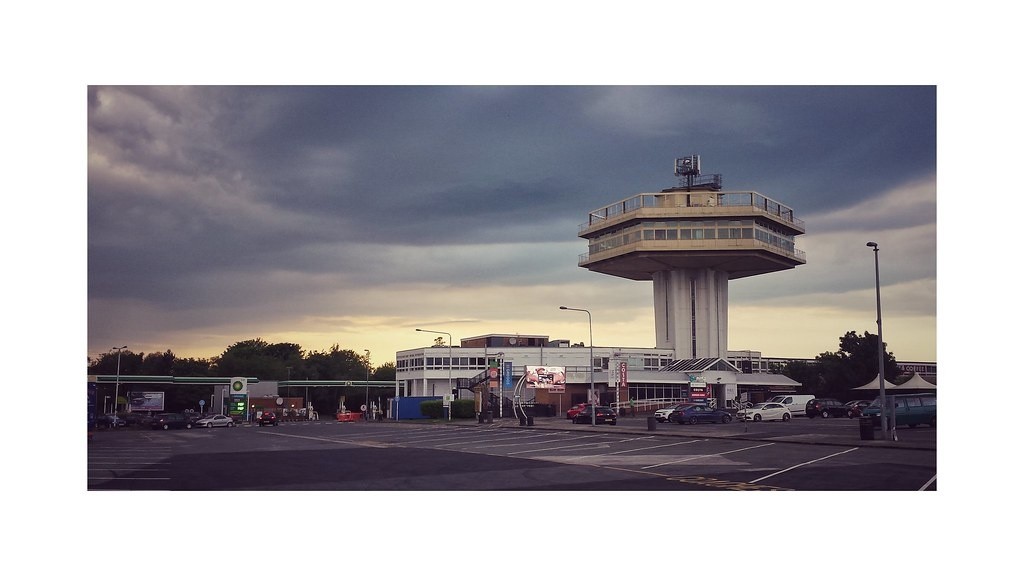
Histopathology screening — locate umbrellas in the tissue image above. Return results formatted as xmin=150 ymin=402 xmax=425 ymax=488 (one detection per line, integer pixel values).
xmin=853 ymin=373 xmax=897 ymax=389
xmin=890 ymin=372 xmax=936 ymax=389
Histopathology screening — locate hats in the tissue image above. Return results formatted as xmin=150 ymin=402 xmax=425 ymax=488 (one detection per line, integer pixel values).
xmin=536 ymin=368 xmax=545 ymax=373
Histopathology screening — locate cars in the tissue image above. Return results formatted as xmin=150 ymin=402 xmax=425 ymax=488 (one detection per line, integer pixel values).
xmin=95 ymin=414 xmax=126 ymax=429
xmin=654 ymin=404 xmax=681 ymax=422
xmin=124 ymin=414 xmax=146 ymax=426
xmin=670 ymin=405 xmax=732 ymax=425
xmin=842 ymin=400 xmax=872 ymax=418
xmin=259 ymin=411 xmax=280 ymax=426
xmin=336 ymin=408 xmax=363 ymax=422
xmin=567 ymin=402 xmax=597 ymax=420
xmin=572 ymin=406 xmax=617 ymax=425
xmin=151 ymin=413 xmax=194 ymax=430
xmin=196 ymin=414 xmax=233 ymax=428
xmin=736 ymin=403 xmax=792 ymax=422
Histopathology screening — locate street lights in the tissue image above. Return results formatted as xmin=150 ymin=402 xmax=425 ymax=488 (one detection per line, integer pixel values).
xmin=867 ymin=242 xmax=887 ymax=437
xmin=559 ymin=306 xmax=595 ymax=426
xmin=365 ymin=349 xmax=369 ymax=420
xmin=416 ymin=329 xmax=452 ymax=421
xmin=113 ymin=345 xmax=128 ymax=427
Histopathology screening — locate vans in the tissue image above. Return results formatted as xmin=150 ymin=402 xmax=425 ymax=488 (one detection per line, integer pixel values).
xmin=770 ymin=395 xmax=815 ymax=415
xmin=805 ymin=399 xmax=844 ymax=418
xmin=861 ymin=393 xmax=936 ymax=428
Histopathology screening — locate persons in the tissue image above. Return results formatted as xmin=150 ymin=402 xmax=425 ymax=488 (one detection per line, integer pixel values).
xmin=630 ymin=396 xmax=636 ymax=418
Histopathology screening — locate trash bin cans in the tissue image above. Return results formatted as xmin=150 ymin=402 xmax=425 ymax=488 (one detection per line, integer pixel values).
xmin=377 ymin=413 xmax=383 ymax=421
xmin=647 ymin=417 xmax=657 ymax=431
xmin=487 ymin=411 xmax=493 ymax=423
xmin=859 ymin=416 xmax=875 ymax=440
